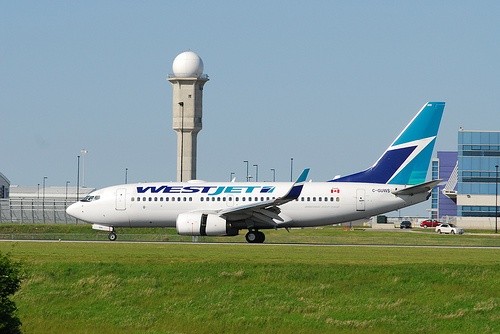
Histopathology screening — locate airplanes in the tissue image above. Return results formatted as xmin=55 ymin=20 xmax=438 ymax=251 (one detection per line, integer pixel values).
xmin=66 ymin=101 xmax=446 ymax=243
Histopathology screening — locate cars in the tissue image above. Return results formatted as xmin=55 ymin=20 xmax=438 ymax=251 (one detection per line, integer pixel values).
xmin=400 ymin=220 xmax=411 ymax=229
xmin=435 ymin=223 xmax=464 ymax=235
xmin=420 ymin=220 xmax=442 ymax=228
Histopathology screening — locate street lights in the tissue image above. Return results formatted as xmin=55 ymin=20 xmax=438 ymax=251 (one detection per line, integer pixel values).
xmin=253 ymin=165 xmax=258 ymax=182
xmin=243 ymin=161 xmax=248 ymax=182
xmin=44 ymin=176 xmax=47 ymax=196
xmin=494 ymin=166 xmax=499 ymax=233
xmin=65 ymin=181 xmax=70 ymax=208
xmin=125 ymin=168 xmax=128 ymax=184
xmin=38 ymin=184 xmax=39 ymax=197
xmin=270 ymin=169 xmax=275 ymax=182
xmin=230 ymin=173 xmax=235 ymax=181
xmin=291 ymin=157 xmax=293 ymax=182
xmin=77 ymin=155 xmax=80 ymax=202
